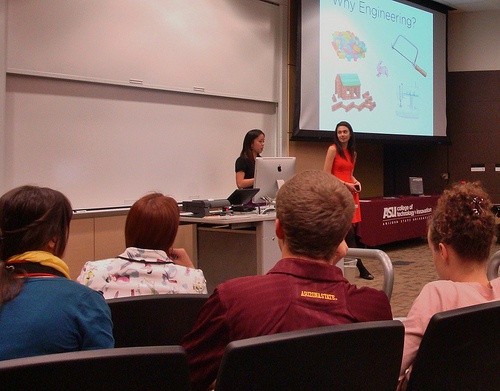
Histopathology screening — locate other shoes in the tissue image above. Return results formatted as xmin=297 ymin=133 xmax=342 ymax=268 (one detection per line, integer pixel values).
xmin=360 ymin=273 xmax=374 ymax=279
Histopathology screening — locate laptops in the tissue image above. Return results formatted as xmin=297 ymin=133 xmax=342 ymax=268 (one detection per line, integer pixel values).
xmin=222 ymin=187 xmax=260 ymax=211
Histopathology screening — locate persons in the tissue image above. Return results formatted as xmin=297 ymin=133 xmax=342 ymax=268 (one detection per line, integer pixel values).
xmin=235 ymin=129 xmax=266 ymax=207
xmin=324 ymin=121 xmax=374 ymax=280
xmin=0 ymin=185 xmax=115 ymax=362
xmin=76 ymin=192 xmax=208 ymax=300
xmin=397 ymin=182 xmax=500 ymax=391
xmin=178 ymin=168 xmax=393 ymax=391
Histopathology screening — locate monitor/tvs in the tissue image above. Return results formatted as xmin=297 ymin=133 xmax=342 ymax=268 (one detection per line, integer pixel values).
xmin=252 ymin=156 xmax=296 ymax=216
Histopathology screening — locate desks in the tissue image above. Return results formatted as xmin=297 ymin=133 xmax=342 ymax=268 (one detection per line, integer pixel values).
xmin=357 ymin=194 xmax=440 ymax=249
xmin=179 ymin=205 xmax=359 ymax=280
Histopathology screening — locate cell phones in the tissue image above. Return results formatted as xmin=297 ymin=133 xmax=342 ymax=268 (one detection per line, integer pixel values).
xmin=355 ymin=185 xmax=359 ymax=191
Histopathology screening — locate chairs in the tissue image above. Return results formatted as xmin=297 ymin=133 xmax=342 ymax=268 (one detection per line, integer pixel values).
xmin=104 ymin=294 xmax=210 ymax=348
xmin=405 ymin=301 xmax=500 ymax=391
xmin=214 ymin=321 xmax=404 ymax=391
xmin=0 ymin=345 xmax=191 ymax=391
